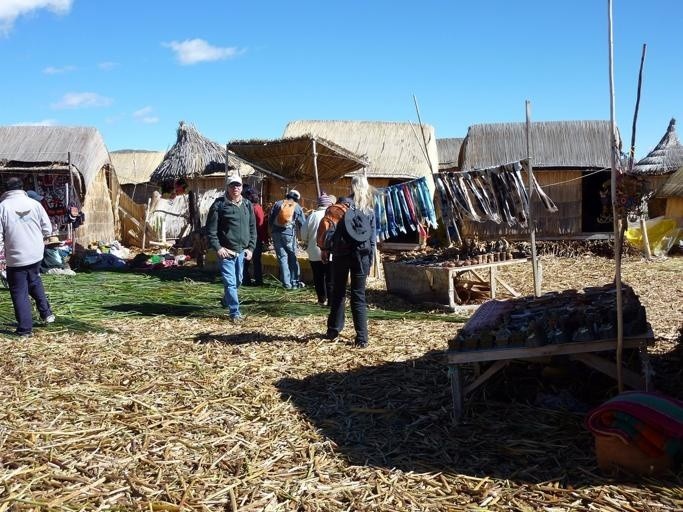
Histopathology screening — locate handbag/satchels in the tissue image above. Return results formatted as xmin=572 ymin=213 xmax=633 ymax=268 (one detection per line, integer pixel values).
xmin=64 ymin=186 xmax=85 ymax=228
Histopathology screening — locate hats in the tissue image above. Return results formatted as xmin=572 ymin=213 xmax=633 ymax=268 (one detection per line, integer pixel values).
xmin=46 ymin=236 xmax=63 ymax=245
xmin=330 ymin=194 xmax=337 ymax=204
xmin=318 ymin=192 xmax=332 ymax=207
xmin=344 ymin=208 xmax=372 ymax=241
xmin=291 ymin=190 xmax=301 ymax=199
xmin=227 ymin=174 xmax=243 ymax=185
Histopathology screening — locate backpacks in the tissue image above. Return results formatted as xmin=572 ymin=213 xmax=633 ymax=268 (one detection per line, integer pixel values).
xmin=314 ymin=203 xmax=350 ymax=250
xmin=278 ymin=200 xmax=296 ymax=225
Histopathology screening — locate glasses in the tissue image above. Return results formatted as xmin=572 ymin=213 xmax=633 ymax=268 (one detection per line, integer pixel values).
xmin=229 ymin=182 xmax=242 ymax=187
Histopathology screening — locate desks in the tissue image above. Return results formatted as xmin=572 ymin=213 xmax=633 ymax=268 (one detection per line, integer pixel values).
xmin=443 ymin=281 xmax=655 ymax=424
xmin=382 ymin=244 xmax=541 ymax=310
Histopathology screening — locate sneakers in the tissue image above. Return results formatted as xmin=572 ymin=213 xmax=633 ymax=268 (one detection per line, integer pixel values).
xmin=45 ymin=313 xmax=56 ymax=324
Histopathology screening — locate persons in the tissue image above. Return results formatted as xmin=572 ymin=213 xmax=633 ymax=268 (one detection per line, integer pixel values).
xmin=203 ymin=174 xmax=376 ymax=348
xmin=0 ymin=178 xmax=56 ymax=336
